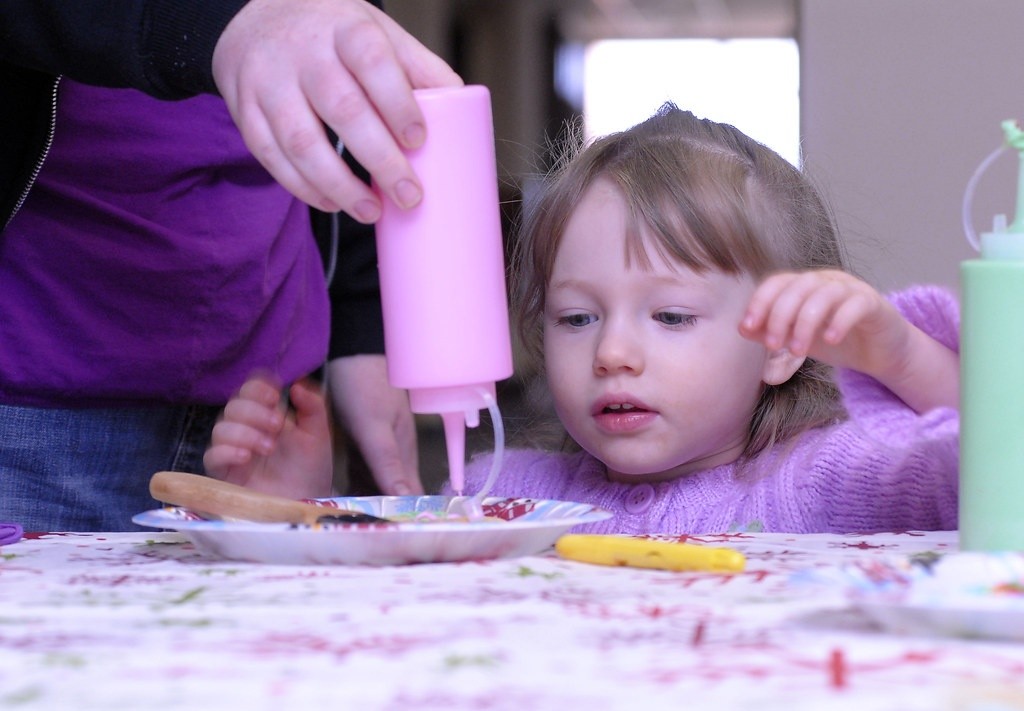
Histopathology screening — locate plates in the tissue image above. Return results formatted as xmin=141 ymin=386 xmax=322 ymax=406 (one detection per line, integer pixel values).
xmin=131 ymin=494 xmax=614 ymax=566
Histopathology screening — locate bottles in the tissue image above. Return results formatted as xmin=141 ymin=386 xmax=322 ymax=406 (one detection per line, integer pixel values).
xmin=958 ymin=120 xmax=1024 ymax=554
xmin=370 ymin=83 xmax=514 ymax=492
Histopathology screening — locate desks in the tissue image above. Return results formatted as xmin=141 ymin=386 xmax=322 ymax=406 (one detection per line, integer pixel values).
xmin=0 ymin=528 xmax=1024 ymax=711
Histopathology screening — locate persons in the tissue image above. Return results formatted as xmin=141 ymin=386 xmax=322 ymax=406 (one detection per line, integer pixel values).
xmin=0 ymin=1 xmax=465 ymax=533
xmin=201 ymin=97 xmax=960 ymax=530
